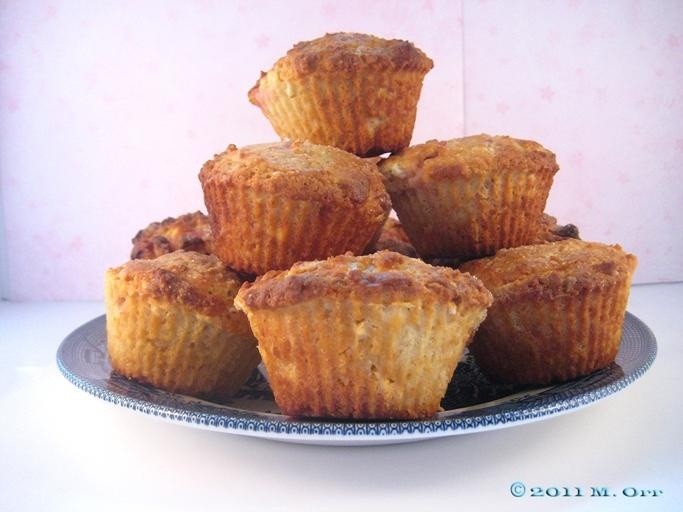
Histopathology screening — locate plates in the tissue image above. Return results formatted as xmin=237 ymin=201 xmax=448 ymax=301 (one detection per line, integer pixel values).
xmin=56 ymin=310 xmax=658 ymax=447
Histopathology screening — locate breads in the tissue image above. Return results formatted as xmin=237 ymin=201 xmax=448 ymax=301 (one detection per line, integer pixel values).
xmin=247 ymin=31 xmax=434 ymax=158
xmin=104 ymin=249 xmax=262 ymax=404
xmin=376 ymin=132 xmax=561 ymax=262
xmin=373 ymin=216 xmax=418 ymax=259
xmin=534 ymin=212 xmax=583 ymax=243
xmin=198 ymin=140 xmax=392 ymax=280
xmin=456 ymin=238 xmax=636 ymax=393
xmin=128 ymin=210 xmax=212 ymax=258
xmin=233 ymin=249 xmax=495 ymax=424
